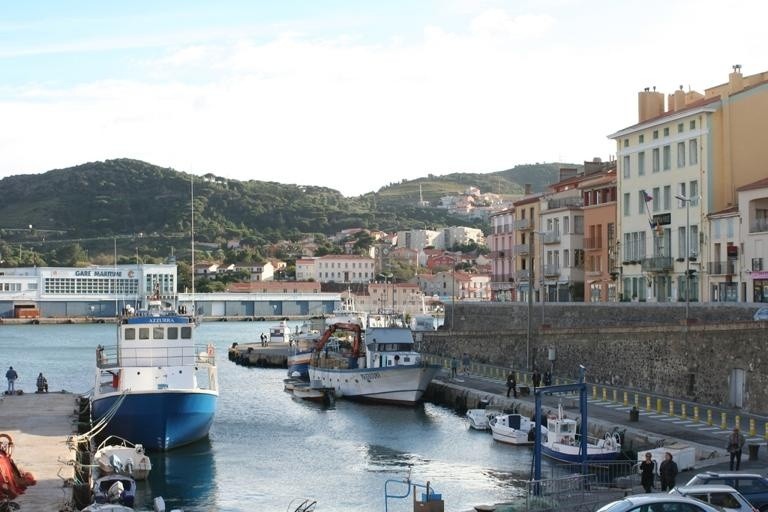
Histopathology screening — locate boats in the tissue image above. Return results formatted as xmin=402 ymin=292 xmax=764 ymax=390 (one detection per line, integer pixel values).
xmin=286 ymin=333 xmax=340 ymax=379
xmin=283 ymin=378 xmax=310 ymax=390
xmin=91 ymin=280 xmax=220 ymax=454
xmin=80 ymin=500 xmax=134 ymax=511
xmin=488 ymin=414 xmax=547 ymax=445
xmin=92 ymin=473 xmax=137 ymax=506
xmin=308 ymin=322 xmax=441 ymax=406
xmin=93 ymin=435 xmax=152 ymax=480
xmin=466 ymin=408 xmax=502 ymax=430
xmin=540 ymin=403 xmax=622 ymax=466
xmin=293 ymin=379 xmax=336 ymax=410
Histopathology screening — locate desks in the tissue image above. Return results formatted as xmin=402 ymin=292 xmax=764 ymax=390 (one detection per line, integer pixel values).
xmin=524 ymin=229 xmax=548 ymax=373
xmin=672 ymin=191 xmax=704 ymax=322
xmin=135 ymin=246 xmax=138 ymax=264
xmin=430 ymin=254 xmax=456 ymax=329
xmin=379 ymin=272 xmax=393 ymax=314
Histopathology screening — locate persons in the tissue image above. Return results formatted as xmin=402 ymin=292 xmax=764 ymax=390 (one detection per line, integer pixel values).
xmin=659 ymin=452 xmax=679 ymax=491
xmin=264 ymin=334 xmax=268 ymax=347
xmin=543 ymin=369 xmax=552 ymax=396
xmin=507 ymin=370 xmax=518 ymax=398
xmin=531 ymin=367 xmax=541 ymax=396
xmin=261 ymin=333 xmax=265 ymax=348
xmin=640 ymin=453 xmax=655 ymax=493
xmin=36 ymin=373 xmax=48 ymax=393
xmin=727 ymin=427 xmax=745 ymax=470
xmin=450 ymin=357 xmax=459 ymax=379
xmin=461 ymin=351 xmax=470 ymax=377
xmin=5 ymin=367 xmax=18 ymax=395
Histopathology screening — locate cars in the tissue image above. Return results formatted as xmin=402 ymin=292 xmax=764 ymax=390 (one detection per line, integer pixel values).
xmin=597 ymin=493 xmax=735 ymax=511
xmin=686 ymin=470 xmax=767 ymax=511
xmin=751 ymin=302 xmax=767 ymax=323
xmin=666 ymin=484 xmax=760 ymax=511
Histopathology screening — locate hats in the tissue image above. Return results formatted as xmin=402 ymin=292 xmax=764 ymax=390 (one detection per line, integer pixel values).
xmin=751 ymin=302 xmax=767 ymax=323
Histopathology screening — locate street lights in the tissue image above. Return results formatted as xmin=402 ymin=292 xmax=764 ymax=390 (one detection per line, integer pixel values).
xmin=135 ymin=246 xmax=138 ymax=264
xmin=672 ymin=191 xmax=704 ymax=322
xmin=524 ymin=229 xmax=548 ymax=373
xmin=430 ymin=254 xmax=456 ymax=329
xmin=379 ymin=272 xmax=393 ymax=314
